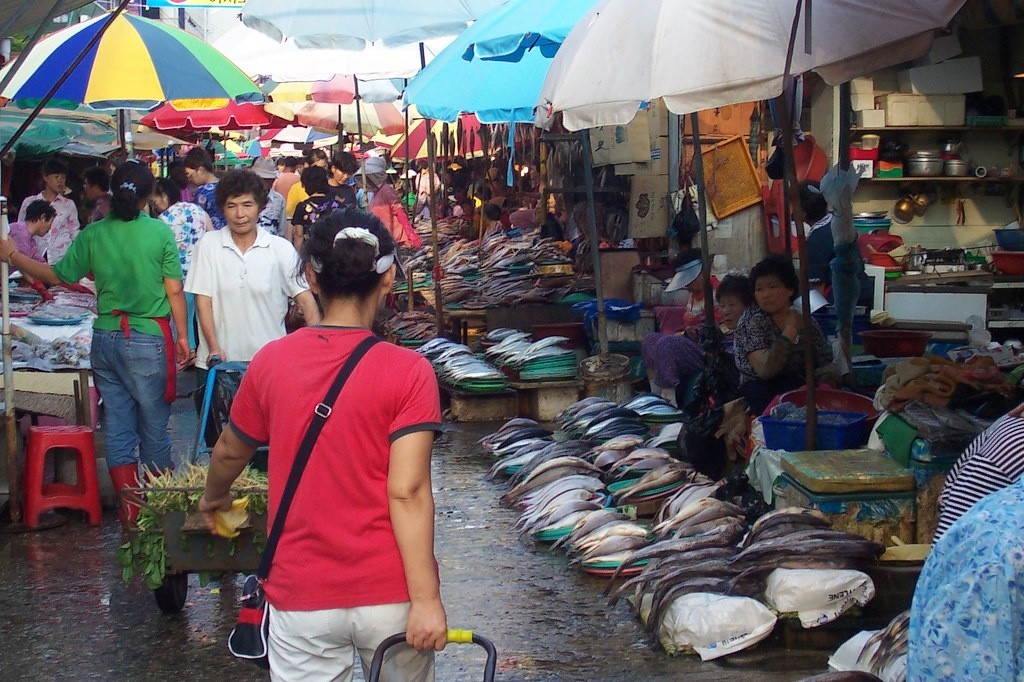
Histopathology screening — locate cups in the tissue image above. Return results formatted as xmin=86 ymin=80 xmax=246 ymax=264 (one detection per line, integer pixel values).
xmin=1008 ymin=110 xmax=1015 ymax=119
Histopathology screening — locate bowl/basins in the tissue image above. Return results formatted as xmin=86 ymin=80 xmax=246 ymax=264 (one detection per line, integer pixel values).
xmin=857 ymin=330 xmax=933 ymax=357
xmin=989 ymin=253 xmax=1024 ymax=274
xmin=943 ymin=160 xmax=967 ymax=176
xmin=992 ymin=228 xmax=1024 ymax=251
xmin=908 ymin=154 xmax=942 ymax=177
xmin=779 ymin=389 xmax=883 ymax=446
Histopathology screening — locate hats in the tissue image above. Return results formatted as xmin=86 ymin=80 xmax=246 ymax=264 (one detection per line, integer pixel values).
xmin=353 ymin=157 xmax=388 ymax=188
xmin=664 ymin=259 xmax=702 ymax=294
xmin=249 ymin=158 xmax=278 ymax=179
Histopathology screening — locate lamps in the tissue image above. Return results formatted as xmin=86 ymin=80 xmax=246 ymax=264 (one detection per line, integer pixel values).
xmin=447 ymin=162 xmax=462 ymax=171
xmin=385 ymin=166 xmax=397 ymax=174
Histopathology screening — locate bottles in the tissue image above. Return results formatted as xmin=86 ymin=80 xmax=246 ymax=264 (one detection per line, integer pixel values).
xmin=911 ymin=244 xmax=920 ymax=265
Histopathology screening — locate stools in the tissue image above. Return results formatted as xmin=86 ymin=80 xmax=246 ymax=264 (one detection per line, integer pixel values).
xmin=22 ymin=424 xmax=103 ymax=529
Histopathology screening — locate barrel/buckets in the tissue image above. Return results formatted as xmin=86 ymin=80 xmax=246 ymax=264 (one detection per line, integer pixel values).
xmin=853 ymin=219 xmax=893 ymax=234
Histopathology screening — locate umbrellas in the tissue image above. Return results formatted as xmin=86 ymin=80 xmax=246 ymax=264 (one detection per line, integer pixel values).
xmin=0 ymin=0 xmax=966 ymax=225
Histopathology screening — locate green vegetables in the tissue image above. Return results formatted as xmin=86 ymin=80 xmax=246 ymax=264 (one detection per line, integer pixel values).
xmin=119 ymin=463 xmax=268 ymax=588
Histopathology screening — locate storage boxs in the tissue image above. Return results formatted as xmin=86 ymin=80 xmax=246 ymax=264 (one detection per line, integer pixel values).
xmin=634 ymin=274 xmax=690 ymax=307
xmin=593 ymin=309 xmax=655 ymax=342
xmin=882 ymin=450 xmax=957 ymax=544
xmin=873 ymin=160 xmax=903 ymax=178
xmin=855 ymin=109 xmax=885 ymax=127
xmin=850 ymin=159 xmax=873 ymax=178
xmin=874 ymin=93 xmax=965 ymax=127
xmin=897 ymin=57 xmax=984 ymax=94
xmin=912 ymin=34 xmax=962 ymax=67
xmin=758 ymin=410 xmax=869 ymax=452
xmin=780 ymin=449 xmax=915 ymax=547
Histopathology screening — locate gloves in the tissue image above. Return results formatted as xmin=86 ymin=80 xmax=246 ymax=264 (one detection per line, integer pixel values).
xmin=61 ymin=281 xmax=95 ymax=295
xmin=31 ymin=279 xmax=53 ymax=303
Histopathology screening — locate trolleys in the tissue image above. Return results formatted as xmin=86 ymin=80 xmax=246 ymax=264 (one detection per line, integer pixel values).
xmin=367 ymin=629 xmax=498 ymax=682
xmin=118 ymin=352 xmax=273 ymax=612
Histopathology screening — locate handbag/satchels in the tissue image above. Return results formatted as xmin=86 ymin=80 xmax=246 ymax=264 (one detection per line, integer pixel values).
xmin=227 ymin=574 xmax=269 ymax=672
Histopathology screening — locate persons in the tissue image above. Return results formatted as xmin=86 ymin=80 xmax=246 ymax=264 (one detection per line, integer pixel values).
xmin=907 ymin=405 xmax=1024 ymax=682
xmin=641 ymin=248 xmax=833 ymax=415
xmin=476 ymin=202 xmax=510 ymax=239
xmin=198 ymin=207 xmax=447 ymax=682
xmin=509 ymin=193 xmax=581 ymax=242
xmin=0 ymin=145 xmax=228 ymax=529
xmin=242 ymin=143 xmax=474 ymax=333
xmin=184 ymin=169 xmax=322 ymax=461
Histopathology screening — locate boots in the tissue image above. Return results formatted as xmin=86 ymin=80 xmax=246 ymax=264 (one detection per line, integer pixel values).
xmin=108 ymin=462 xmax=142 ymax=527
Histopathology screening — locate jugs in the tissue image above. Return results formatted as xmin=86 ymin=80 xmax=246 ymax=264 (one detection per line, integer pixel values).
xmin=895 ymin=193 xmax=928 ymax=220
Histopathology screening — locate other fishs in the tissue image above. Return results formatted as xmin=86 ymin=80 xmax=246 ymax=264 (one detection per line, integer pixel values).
xmin=357 ymin=201 xmax=911 ymax=682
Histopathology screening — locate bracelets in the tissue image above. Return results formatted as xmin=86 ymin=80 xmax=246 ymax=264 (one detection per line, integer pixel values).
xmin=8 ymin=250 xmax=20 ymax=266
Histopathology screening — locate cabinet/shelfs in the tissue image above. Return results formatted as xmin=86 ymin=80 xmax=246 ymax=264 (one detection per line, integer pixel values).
xmin=849 ymin=125 xmax=1024 ymax=180
